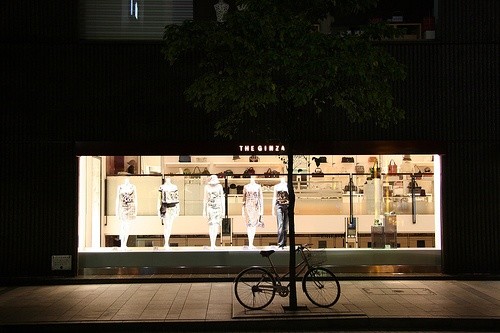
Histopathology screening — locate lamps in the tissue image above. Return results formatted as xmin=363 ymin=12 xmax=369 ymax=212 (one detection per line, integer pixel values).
xmin=123 ymin=1 xmax=144 ymax=25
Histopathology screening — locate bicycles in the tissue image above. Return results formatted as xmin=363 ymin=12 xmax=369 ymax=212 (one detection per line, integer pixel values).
xmin=234 ymin=243 xmax=341 ymax=310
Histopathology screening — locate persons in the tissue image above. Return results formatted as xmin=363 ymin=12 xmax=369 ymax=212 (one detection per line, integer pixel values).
xmin=203 ymin=175 xmax=225 ymax=250
xmin=272 ymin=178 xmax=295 ymax=250
xmin=157 ymin=178 xmax=179 ymax=251
xmin=116 ymin=178 xmax=138 ymax=251
xmin=241 ymin=178 xmax=264 ymax=251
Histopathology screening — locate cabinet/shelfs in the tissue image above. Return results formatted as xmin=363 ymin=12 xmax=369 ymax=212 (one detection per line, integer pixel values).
xmin=95 ymin=155 xmax=435 ymax=251
